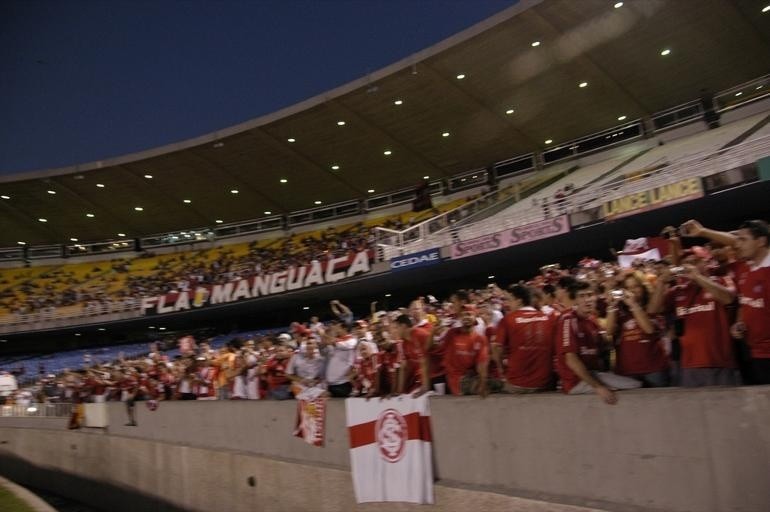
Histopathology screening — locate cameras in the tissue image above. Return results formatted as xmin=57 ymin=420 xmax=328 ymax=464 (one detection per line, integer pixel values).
xmin=611 ymin=290 xmax=624 ymax=299
xmin=663 ymin=224 xmax=688 ymax=239
xmin=671 ymin=266 xmax=686 ymax=276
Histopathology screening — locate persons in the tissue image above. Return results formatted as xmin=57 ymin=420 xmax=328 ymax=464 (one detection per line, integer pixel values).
xmin=2 ymin=175 xmax=501 ymax=317
xmin=1 ymin=284 xmax=507 ymax=403
xmin=496 ymin=217 xmax=770 ymax=405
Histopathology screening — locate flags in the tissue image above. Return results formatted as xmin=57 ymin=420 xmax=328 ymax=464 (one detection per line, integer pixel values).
xmin=292 ymin=396 xmax=326 ymax=450
xmin=340 ymin=391 xmax=438 ymax=504
xmin=67 ymin=401 xmax=110 ymax=431
